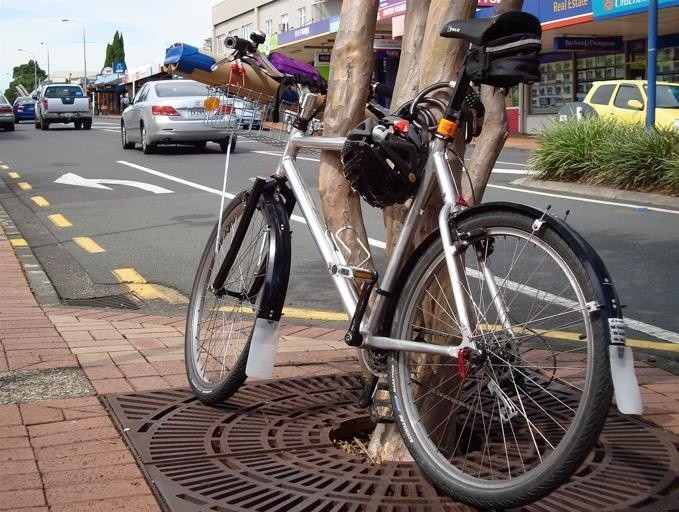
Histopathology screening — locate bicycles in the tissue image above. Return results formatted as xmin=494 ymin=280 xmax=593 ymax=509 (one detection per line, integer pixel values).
xmin=162 ymin=10 xmax=645 ymax=509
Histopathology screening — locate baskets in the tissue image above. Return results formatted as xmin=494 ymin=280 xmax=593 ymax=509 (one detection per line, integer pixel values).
xmin=204 ymin=84 xmax=324 ymax=156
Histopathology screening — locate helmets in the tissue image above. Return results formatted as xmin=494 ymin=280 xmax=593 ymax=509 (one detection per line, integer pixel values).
xmin=343 ymin=115 xmax=429 ymax=210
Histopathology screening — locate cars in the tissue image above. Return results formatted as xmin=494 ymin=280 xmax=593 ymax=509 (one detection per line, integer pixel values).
xmin=1 ymin=95 xmax=16 ymax=132
xmin=228 ymin=97 xmax=262 ymax=131
xmin=119 ymin=80 xmax=237 ymax=154
xmin=582 ymin=76 xmax=679 ymax=136
xmin=12 ymin=95 xmax=34 ymax=124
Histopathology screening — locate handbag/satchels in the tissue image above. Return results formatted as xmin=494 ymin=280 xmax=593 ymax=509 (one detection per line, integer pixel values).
xmin=255 ymin=52 xmax=323 ymax=81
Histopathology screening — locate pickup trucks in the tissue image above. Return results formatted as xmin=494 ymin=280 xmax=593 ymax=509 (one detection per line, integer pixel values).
xmin=32 ymin=84 xmax=93 ymax=130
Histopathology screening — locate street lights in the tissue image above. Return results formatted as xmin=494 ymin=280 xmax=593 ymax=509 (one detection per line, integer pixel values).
xmin=41 ymin=42 xmax=51 ymax=84
xmin=19 ymin=48 xmax=38 ymax=90
xmin=62 ymin=18 xmax=87 ymax=96
xmin=6 ymin=73 xmax=12 ymax=81
